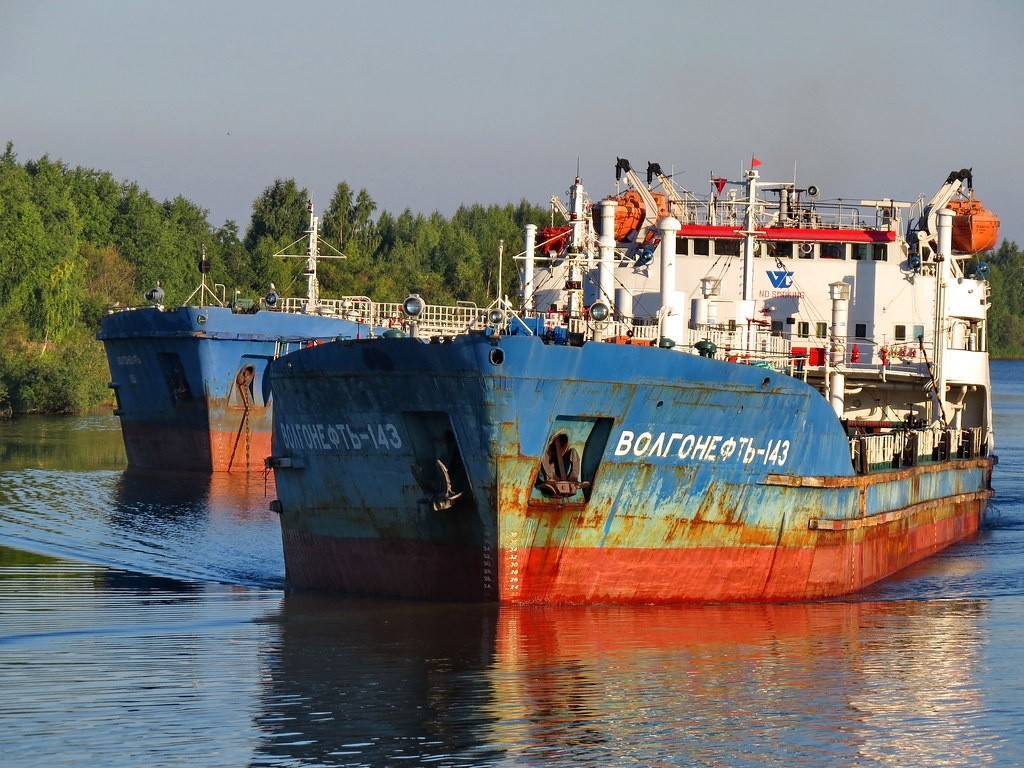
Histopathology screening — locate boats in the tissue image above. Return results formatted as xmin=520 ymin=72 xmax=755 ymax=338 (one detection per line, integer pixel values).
xmin=94 ymin=189 xmax=540 ymax=473
xmin=260 ymin=151 xmax=1003 ymax=612
